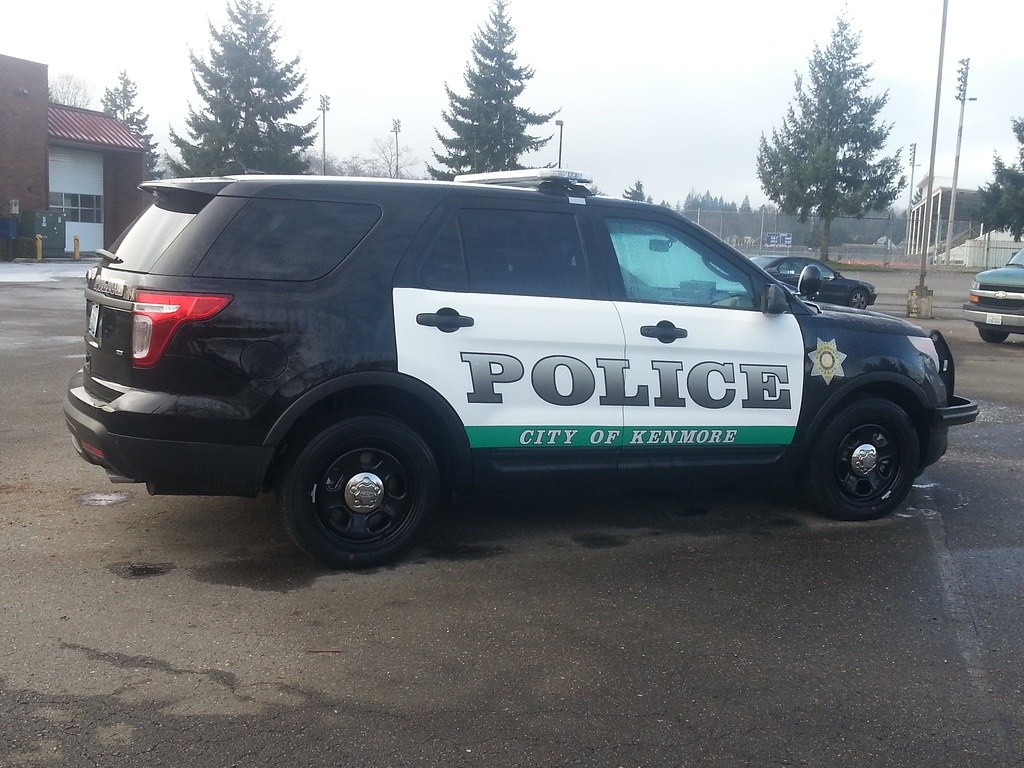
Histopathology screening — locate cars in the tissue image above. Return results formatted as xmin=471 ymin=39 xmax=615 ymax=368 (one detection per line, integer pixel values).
xmin=715 ymin=254 xmax=877 ymax=314
xmin=963 ymin=247 xmax=1024 ymax=343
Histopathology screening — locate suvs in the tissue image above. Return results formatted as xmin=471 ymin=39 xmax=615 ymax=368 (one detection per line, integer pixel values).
xmin=62 ymin=168 xmax=982 ymax=569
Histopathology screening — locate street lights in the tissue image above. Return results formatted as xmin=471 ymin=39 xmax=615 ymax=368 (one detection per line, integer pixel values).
xmin=317 ymin=94 xmax=331 ymax=174
xmin=943 ymin=57 xmax=970 ymax=264
xmin=905 ymin=142 xmax=917 ymax=256
xmin=391 ymin=117 xmax=402 ymax=178
xmin=556 ymin=120 xmax=564 ymax=169
